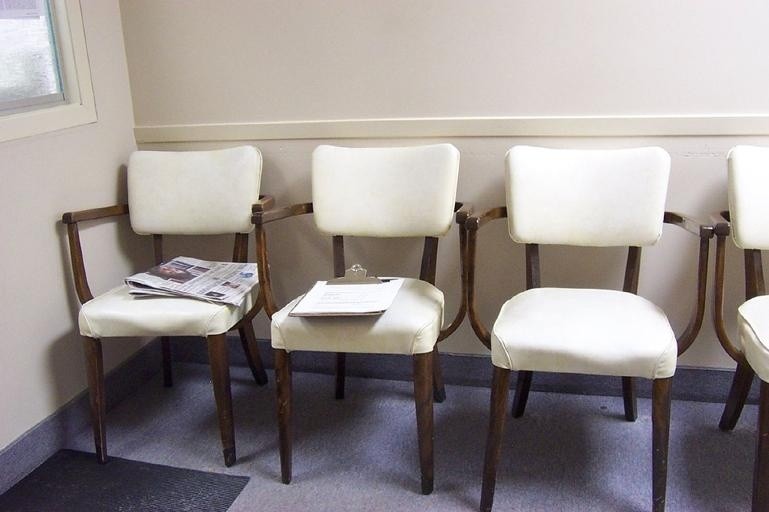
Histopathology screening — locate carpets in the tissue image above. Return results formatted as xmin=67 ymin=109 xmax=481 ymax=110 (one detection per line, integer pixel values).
xmin=0 ymin=448 xmax=249 ymax=512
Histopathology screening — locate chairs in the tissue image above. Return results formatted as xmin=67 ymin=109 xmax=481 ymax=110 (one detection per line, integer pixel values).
xmin=250 ymin=143 xmax=473 ymax=497
xmin=710 ymin=145 xmax=769 ymax=512
xmin=62 ymin=144 xmax=274 ymax=468
xmin=464 ymin=144 xmax=715 ymax=512
